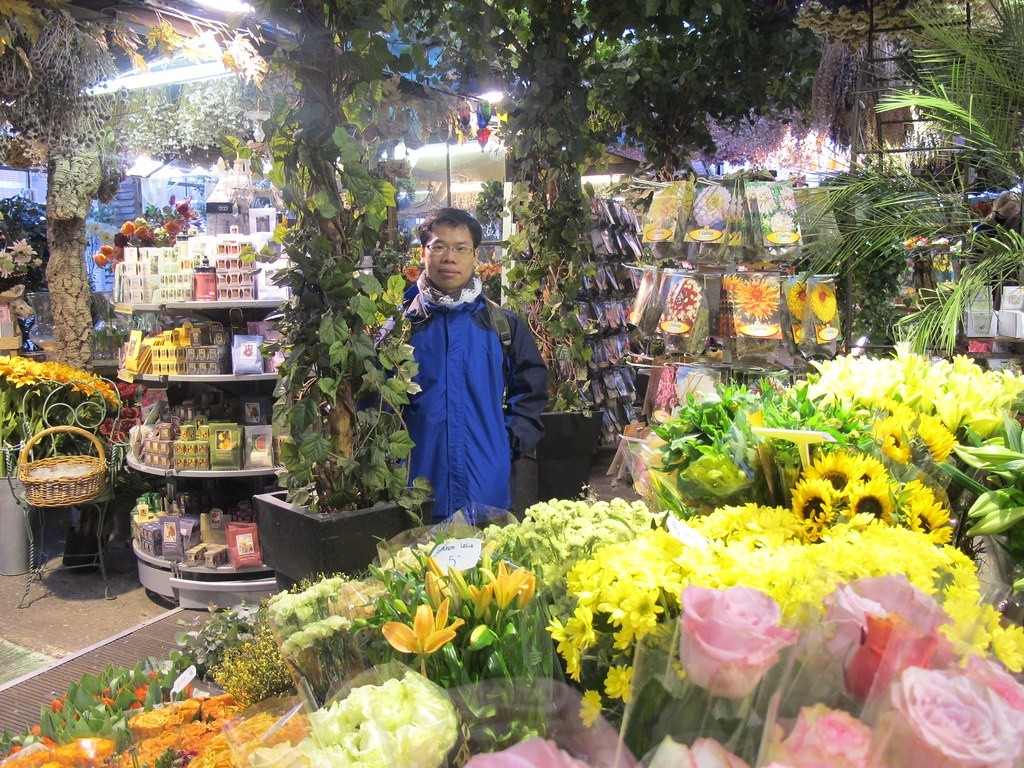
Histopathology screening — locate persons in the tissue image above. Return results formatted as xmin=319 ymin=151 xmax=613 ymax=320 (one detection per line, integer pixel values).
xmin=374 ymin=208 xmax=547 ymax=532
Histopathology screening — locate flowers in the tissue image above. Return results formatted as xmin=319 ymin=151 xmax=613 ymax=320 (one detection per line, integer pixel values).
xmin=86 ymin=220 xmax=118 ymax=246
xmin=0 ymin=195 xmax=47 ymax=304
xmin=94 ymin=194 xmax=203 ymax=271
xmin=0 ymin=350 xmax=1024 ymax=767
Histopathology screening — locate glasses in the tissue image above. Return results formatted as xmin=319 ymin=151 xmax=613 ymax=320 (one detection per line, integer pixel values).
xmin=424 ymin=244 xmax=475 ymax=257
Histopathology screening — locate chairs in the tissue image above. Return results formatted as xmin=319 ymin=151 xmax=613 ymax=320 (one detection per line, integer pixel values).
xmin=0 ymin=380 xmax=130 ymax=609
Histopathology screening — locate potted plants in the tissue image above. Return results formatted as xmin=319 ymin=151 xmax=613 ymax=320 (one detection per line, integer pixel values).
xmin=252 ymin=0 xmax=801 ymax=599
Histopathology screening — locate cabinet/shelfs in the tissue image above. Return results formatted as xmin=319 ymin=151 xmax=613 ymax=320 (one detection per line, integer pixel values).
xmin=114 ymin=232 xmax=296 ymax=610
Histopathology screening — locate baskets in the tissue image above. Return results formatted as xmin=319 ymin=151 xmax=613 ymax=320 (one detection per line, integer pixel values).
xmin=20 ymin=425 xmax=110 ymax=508
